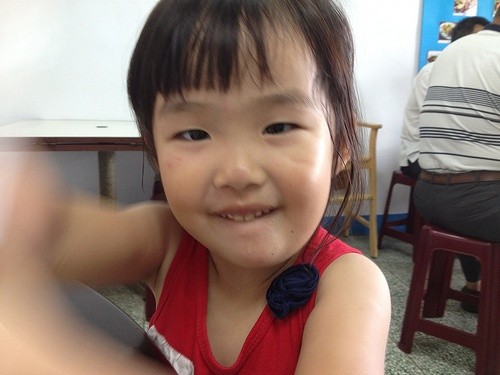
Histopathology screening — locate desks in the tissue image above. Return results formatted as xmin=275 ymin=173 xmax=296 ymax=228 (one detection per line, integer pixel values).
xmin=0 ymin=119 xmax=153 ymax=290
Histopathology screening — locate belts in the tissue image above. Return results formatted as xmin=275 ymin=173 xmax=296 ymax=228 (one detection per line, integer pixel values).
xmin=419 ymin=169 xmax=500 ymax=184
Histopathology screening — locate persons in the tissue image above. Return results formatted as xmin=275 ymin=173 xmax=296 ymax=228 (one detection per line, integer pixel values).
xmin=414 ymin=0 xmax=500 ymax=313
xmin=0 ymin=0 xmax=391 ymax=375
xmin=399 ymin=16 xmax=489 ymax=176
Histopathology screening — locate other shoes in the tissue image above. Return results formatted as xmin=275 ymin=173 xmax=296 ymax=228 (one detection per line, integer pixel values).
xmin=460 ymin=285 xmax=482 ymax=314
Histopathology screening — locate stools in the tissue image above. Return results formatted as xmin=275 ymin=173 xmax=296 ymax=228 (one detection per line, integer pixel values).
xmin=397 ymin=224 xmax=500 ymax=375
xmin=377 ymin=169 xmax=431 ymax=263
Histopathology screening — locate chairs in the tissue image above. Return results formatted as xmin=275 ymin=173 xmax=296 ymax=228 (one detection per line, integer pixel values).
xmin=328 ymin=122 xmax=382 ymax=259
